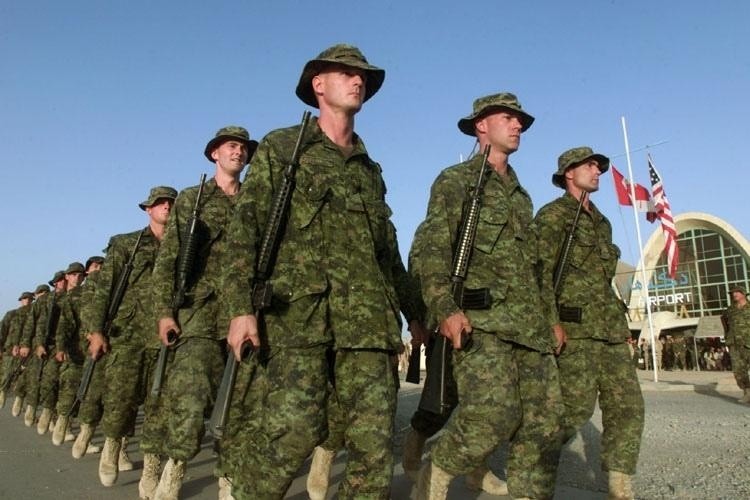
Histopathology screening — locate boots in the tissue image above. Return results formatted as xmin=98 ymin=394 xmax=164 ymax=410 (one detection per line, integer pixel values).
xmin=409 ymin=461 xmax=455 ymax=500
xmin=71 ymin=424 xmax=102 ymax=459
xmin=402 ymin=429 xmax=425 ymax=481
xmin=98 ymin=435 xmax=133 ymax=487
xmin=465 ymin=465 xmax=508 ymax=496
xmin=738 ymin=388 xmax=750 ymax=403
xmin=608 ymin=470 xmax=634 ymax=500
xmin=0 ymin=390 xmax=75 ymax=446
xmin=306 ymin=446 xmax=336 ymax=500
xmin=138 ymin=453 xmax=186 ymax=500
xmin=218 ymin=476 xmax=235 ymax=500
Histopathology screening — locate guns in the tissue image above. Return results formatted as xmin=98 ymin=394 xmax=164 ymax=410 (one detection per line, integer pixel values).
xmin=151 ymin=173 xmax=208 ymax=400
xmin=418 ymin=144 xmax=491 ymax=415
xmin=555 ymin=190 xmax=587 ymax=323
xmin=37 ymin=282 xmax=60 ymax=381
xmin=208 ymin=108 xmax=312 ymax=439
xmin=76 ymin=230 xmax=144 ymax=402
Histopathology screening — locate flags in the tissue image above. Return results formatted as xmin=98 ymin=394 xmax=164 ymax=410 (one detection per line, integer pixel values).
xmin=610 ymin=160 xmax=680 ymax=280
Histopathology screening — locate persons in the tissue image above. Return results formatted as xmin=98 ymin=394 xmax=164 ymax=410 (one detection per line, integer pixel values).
xmin=630 ymin=336 xmax=732 ymax=372
xmin=725 ymin=286 xmax=750 ymax=404
xmin=2 ymin=44 xmax=645 ymax=499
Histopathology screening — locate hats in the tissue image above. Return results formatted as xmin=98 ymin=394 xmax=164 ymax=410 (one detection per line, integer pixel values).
xmin=18 ymin=256 xmax=104 ymax=301
xmin=139 ymin=186 xmax=177 ymax=211
xmin=295 ymin=43 xmax=385 ymax=109
xmin=552 ymin=147 xmax=610 ymax=189
xmin=727 ymin=286 xmax=747 ymax=295
xmin=457 ymin=93 xmax=535 ymax=137
xmin=204 ymin=125 xmax=259 ymax=164
